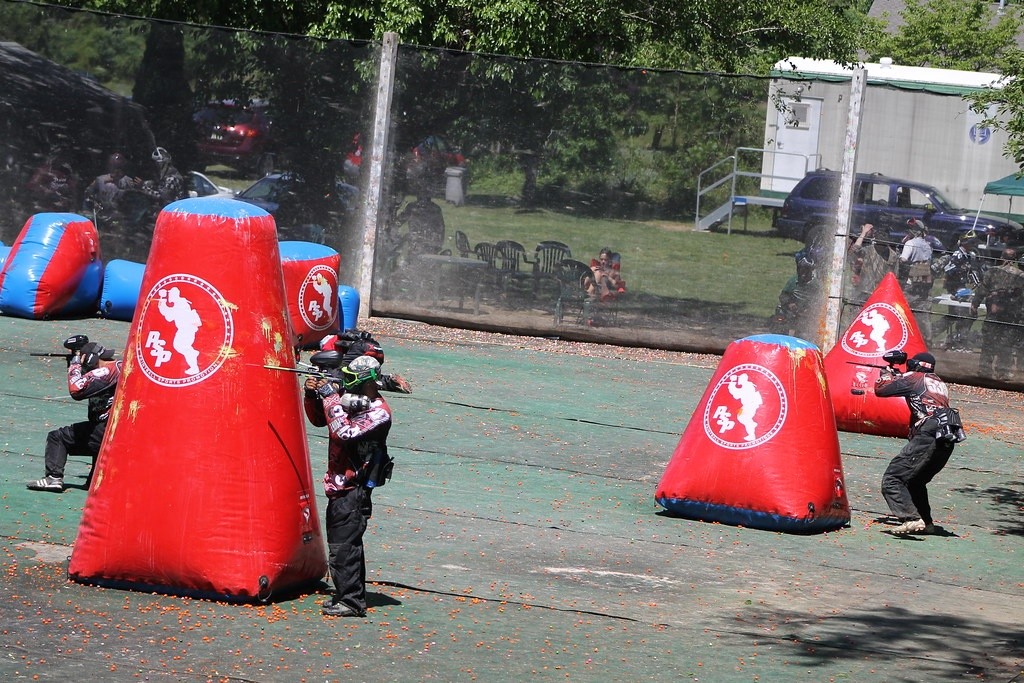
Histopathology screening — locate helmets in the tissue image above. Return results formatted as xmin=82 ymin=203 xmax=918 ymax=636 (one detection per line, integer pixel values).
xmin=908 ymin=219 xmax=924 ymax=239
xmin=349 ymin=356 xmax=381 ymax=383
xmin=151 ymin=147 xmax=172 ymax=165
xmin=107 ymin=153 xmax=129 ymax=171
xmin=80 ymin=343 xmax=115 ymax=370
xmin=910 ymin=353 xmax=935 ymax=373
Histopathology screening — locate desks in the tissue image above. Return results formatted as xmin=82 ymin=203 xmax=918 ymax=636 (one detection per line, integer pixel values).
xmin=417 ymin=253 xmax=489 ymax=315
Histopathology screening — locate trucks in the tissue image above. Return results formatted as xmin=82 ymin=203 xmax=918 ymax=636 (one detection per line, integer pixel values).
xmin=759 ymin=54 xmax=1024 ymax=228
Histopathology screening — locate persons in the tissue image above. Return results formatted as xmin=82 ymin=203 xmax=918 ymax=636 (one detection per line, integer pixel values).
xmin=304 ymin=330 xmax=394 ymax=617
xmin=583 ymin=248 xmax=620 ymax=325
xmin=27 ymin=341 xmax=122 ymax=492
xmin=874 ymin=352 xmax=966 ymax=535
xmin=0 ymin=101 xmax=185 ymax=263
xmin=772 ymin=219 xmax=1024 ymax=378
xmin=343 ymin=130 xmax=367 ymax=213
xmin=413 ymin=139 xmax=464 ymax=168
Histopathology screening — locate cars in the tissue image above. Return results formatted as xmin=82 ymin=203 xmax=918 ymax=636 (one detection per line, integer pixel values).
xmin=136 ymin=97 xmax=465 ymax=301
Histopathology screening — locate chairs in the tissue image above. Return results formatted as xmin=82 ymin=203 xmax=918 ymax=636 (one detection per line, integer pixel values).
xmin=453 ymin=230 xmax=624 ymax=328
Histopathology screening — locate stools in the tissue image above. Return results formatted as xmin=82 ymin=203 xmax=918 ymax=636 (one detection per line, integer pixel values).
xmin=70 ymin=452 xmax=98 ymax=490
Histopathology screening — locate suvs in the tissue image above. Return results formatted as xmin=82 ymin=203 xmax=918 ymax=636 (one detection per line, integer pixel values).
xmin=772 ymin=166 xmax=1024 ymax=265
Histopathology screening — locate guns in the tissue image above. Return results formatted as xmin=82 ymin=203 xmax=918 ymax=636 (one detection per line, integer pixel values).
xmin=85 ymin=193 xmax=102 ymax=229
xmin=29 ymin=335 xmax=88 ymax=373
xmin=263 ymin=349 xmax=343 ymax=388
xmin=845 ymin=350 xmax=908 ymax=376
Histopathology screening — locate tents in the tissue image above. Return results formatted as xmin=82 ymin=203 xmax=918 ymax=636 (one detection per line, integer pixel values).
xmin=973 ymin=172 xmax=1024 ymax=229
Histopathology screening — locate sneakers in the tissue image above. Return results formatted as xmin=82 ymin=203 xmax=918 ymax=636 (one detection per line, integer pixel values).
xmin=323 ymin=599 xmax=332 ymax=606
xmin=26 ymin=475 xmax=63 ymax=491
xmin=322 ymin=600 xmax=357 ymax=616
xmin=892 ymin=518 xmax=926 ymax=534
xmin=922 ymin=522 xmax=934 ymax=534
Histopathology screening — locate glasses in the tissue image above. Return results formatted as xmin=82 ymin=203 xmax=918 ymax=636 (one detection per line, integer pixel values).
xmin=341 ymin=366 xmax=360 ymax=389
xmin=908 ymin=217 xmax=916 ymax=225
xmin=907 ymin=359 xmax=917 ymax=369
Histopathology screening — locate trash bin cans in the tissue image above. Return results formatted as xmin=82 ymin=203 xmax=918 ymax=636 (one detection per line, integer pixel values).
xmin=444 ymin=167 xmax=467 ymax=203
